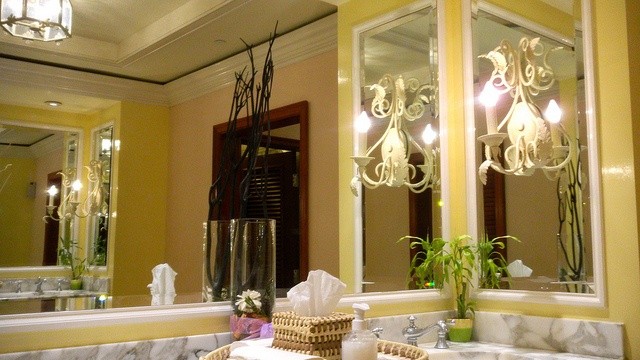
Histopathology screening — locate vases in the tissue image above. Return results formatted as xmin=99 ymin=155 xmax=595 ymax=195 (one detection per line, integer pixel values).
xmin=248 ymin=316 xmax=270 ymax=336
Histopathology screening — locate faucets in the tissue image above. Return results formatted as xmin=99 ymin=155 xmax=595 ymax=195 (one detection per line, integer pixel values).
xmin=401 ymin=315 xmax=448 ymax=347
xmin=34 ymin=276 xmax=47 ymax=294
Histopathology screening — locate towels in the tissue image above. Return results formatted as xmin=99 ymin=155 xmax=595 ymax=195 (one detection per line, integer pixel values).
xmin=229 ymin=345 xmax=326 ymax=360
xmin=229 ymin=335 xmax=275 ymax=351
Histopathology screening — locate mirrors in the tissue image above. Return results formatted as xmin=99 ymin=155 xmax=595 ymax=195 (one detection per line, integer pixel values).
xmin=59 ymin=134 xmax=82 ymax=264
xmin=0 ymin=0 xmax=340 ymax=317
xmin=88 ymin=121 xmax=117 ymax=272
xmin=0 ymin=118 xmax=84 ymax=273
xmin=351 ymin=0 xmax=448 ymax=297
xmin=461 ymin=0 xmax=609 ymax=307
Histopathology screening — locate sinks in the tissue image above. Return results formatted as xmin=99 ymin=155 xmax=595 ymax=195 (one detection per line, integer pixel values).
xmin=426 ymin=351 xmax=532 ymax=360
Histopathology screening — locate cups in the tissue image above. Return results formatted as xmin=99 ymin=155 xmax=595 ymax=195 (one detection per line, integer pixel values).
xmin=200 ymin=220 xmax=229 ymax=301
xmin=558 ymin=235 xmax=586 ymax=282
xmin=229 ymin=217 xmax=277 ymax=315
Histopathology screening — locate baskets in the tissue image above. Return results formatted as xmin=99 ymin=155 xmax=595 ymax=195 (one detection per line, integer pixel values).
xmin=201 ymin=331 xmax=430 ymax=360
xmin=272 ymin=312 xmax=354 ymax=359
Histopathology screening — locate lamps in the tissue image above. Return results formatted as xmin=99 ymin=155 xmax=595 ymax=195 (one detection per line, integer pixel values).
xmin=0 ymin=0 xmax=72 ymax=43
xmin=475 ymin=36 xmax=572 ymax=188
xmin=350 ymin=73 xmax=441 ymax=198
xmin=70 ymin=157 xmax=111 ymax=217
xmin=42 ymin=158 xmax=77 ymax=225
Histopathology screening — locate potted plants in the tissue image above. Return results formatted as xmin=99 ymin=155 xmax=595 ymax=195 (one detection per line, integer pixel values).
xmin=93 ymin=235 xmax=107 ymax=265
xmin=57 ymin=237 xmax=84 ymax=266
xmin=417 ymin=233 xmax=500 ymax=341
xmin=70 ymin=261 xmax=87 ymax=290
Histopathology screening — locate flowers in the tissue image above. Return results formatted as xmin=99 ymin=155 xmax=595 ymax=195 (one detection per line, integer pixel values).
xmin=235 ymin=290 xmax=262 ymax=313
